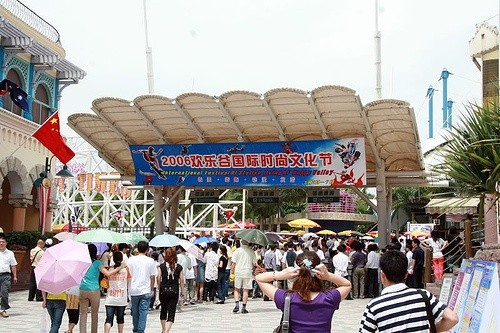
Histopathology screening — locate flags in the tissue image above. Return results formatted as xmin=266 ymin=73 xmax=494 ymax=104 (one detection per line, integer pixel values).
xmin=0 ymin=78 xmax=32 ymax=113
xmin=32 ymin=112 xmax=76 ymax=164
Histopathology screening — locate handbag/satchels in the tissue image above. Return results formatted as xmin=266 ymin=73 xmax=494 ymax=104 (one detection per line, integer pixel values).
xmin=272 ymin=292 xmax=291 ymax=333
xmin=100 ymin=266 xmax=111 ymax=288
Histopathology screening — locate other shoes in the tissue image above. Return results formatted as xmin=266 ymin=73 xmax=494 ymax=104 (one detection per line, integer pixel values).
xmin=184 ymin=304 xmax=188 ymax=306
xmin=203 ymin=301 xmax=207 ymax=305
xmin=155 ymin=301 xmax=161 ymax=309
xmin=191 ymin=302 xmax=196 ymax=305
xmin=215 ymin=300 xmax=224 ymax=304
xmin=241 ymin=309 xmax=248 ymax=313
xmin=211 ymin=301 xmax=215 ymax=304
xmin=0 ymin=310 xmax=9 ymax=316
xmin=233 ymin=308 xmax=239 ymax=313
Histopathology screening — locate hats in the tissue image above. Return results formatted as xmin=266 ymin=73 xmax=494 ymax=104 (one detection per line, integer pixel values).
xmin=45 ymin=239 xmax=52 ymax=245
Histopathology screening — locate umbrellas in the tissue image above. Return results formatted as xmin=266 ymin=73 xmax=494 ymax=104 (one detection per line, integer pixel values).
xmin=230 ymin=228 xmax=273 ymax=247
xmin=192 ymin=235 xmax=219 ymax=244
xmin=148 ymin=234 xmax=182 ymax=247
xmin=33 ymin=240 xmax=92 ymax=294
xmin=266 ymin=218 xmax=379 ymax=240
xmin=73 ymin=229 xmax=133 ymax=245
xmin=53 ymin=231 xmax=77 ymax=242
xmin=121 ymin=232 xmax=150 ymax=244
xmin=179 ymin=239 xmax=205 ymax=263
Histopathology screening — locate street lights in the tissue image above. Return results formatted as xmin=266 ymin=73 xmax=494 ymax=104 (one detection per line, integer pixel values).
xmin=33 ymin=155 xmax=73 ymax=235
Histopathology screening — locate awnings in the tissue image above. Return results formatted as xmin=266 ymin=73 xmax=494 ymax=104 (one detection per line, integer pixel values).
xmin=424 ymin=196 xmax=480 ymax=214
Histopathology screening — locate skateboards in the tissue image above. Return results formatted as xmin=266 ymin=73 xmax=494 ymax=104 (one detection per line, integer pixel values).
xmin=150 ymin=163 xmax=166 ymax=179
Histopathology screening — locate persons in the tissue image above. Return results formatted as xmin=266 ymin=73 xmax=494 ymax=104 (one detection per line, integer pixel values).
xmin=28 ymin=234 xmax=462 ymax=333
xmin=157 ymin=248 xmax=185 ymax=333
xmin=441 ymin=226 xmax=462 ymax=270
xmin=423 ymin=229 xmax=447 ymax=282
xmin=359 ymin=250 xmax=460 ymax=333
xmin=255 ymin=252 xmax=353 ymax=333
xmin=0 ymin=236 xmax=18 ymax=317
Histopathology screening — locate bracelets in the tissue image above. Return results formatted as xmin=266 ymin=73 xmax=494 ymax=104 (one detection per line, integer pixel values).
xmin=273 ymin=270 xmax=277 ymax=281
